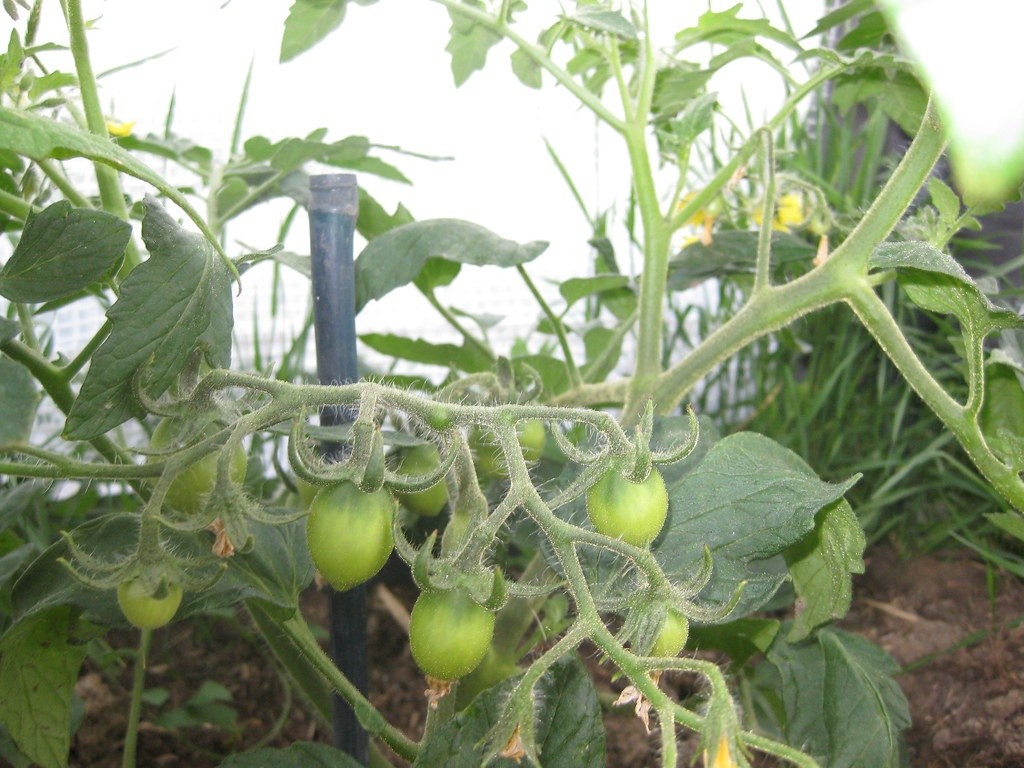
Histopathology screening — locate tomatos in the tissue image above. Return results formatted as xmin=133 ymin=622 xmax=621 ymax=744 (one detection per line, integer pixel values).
xmin=118 ymin=393 xmax=690 ymax=680
